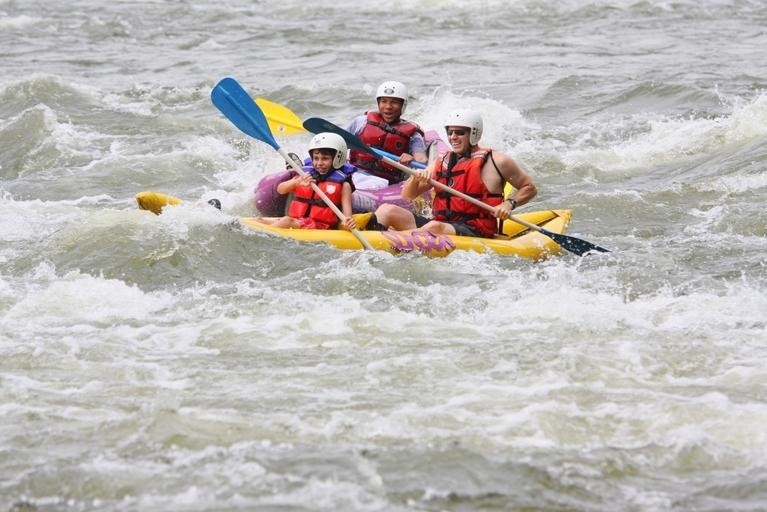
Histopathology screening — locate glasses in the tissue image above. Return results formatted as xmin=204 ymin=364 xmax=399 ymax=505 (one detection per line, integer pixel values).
xmin=448 ymin=130 xmax=464 ymax=135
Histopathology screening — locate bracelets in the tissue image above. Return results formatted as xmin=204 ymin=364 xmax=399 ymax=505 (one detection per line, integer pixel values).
xmin=506 ymin=198 xmax=516 ymax=210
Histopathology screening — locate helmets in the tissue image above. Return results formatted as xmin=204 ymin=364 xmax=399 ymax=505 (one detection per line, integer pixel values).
xmin=308 ymin=133 xmax=347 ymax=169
xmin=376 ymin=81 xmax=407 ymax=115
xmin=444 ymin=110 xmax=483 ymax=145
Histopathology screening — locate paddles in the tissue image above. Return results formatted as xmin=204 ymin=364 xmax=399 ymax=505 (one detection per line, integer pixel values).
xmin=254 ymin=98 xmax=427 ymax=171
xmin=303 ymin=118 xmax=611 ymax=258
xmin=211 ymin=77 xmax=374 ymax=250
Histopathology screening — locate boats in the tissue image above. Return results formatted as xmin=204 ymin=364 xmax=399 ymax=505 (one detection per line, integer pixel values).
xmin=135 ymin=189 xmax=573 ymax=262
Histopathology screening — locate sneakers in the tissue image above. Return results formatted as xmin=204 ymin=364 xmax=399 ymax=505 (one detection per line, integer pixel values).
xmin=286 ymin=153 xmax=303 ymax=168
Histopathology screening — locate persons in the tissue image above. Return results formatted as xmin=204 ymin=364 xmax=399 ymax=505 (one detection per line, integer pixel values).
xmin=342 ymin=81 xmax=428 ymax=191
xmin=255 ymin=131 xmax=359 ymax=233
xmin=359 ymin=109 xmax=537 ymax=239
